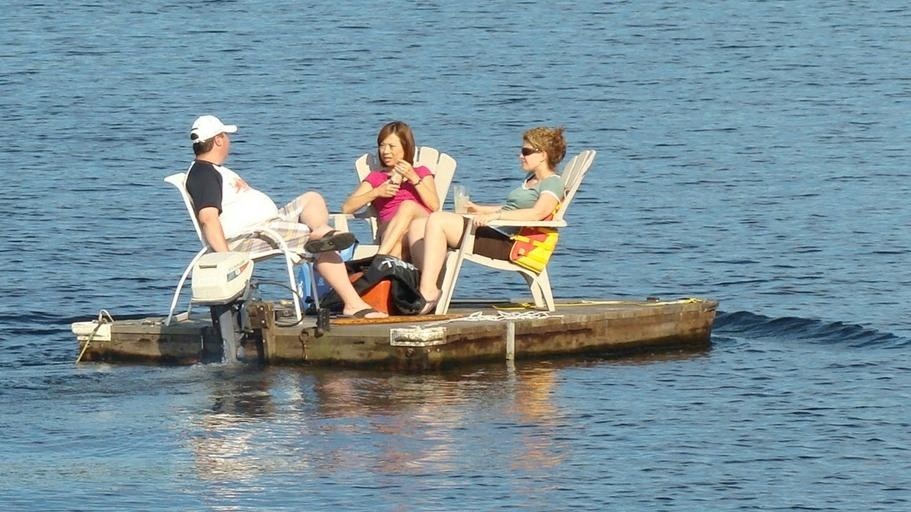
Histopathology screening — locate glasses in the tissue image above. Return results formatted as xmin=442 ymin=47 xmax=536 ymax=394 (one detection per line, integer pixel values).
xmin=522 ymin=147 xmax=543 ymax=156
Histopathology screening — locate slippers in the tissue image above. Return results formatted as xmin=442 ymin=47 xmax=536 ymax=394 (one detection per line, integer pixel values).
xmin=336 ymin=307 xmax=379 ymax=321
xmin=420 ymin=290 xmax=442 ymax=314
xmin=304 ymin=230 xmax=354 ymax=253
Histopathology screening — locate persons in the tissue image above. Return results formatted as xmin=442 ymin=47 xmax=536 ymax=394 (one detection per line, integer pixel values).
xmin=407 ymin=125 xmax=568 ymax=316
xmin=340 ymin=120 xmax=442 ymax=261
xmin=181 ymin=113 xmax=388 ymax=321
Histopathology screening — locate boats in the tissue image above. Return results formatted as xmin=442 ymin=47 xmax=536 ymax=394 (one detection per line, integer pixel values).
xmin=71 ymin=293 xmax=717 ymax=370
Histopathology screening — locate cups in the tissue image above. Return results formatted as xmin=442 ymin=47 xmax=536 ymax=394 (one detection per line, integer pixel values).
xmin=453 ymin=184 xmax=470 ymax=215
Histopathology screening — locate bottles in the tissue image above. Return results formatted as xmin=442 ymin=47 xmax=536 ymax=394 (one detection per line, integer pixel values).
xmin=389 ymin=167 xmax=403 ymax=187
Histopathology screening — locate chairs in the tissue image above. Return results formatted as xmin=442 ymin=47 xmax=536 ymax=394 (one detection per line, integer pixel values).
xmin=333 ymin=145 xmax=459 ymax=259
xmin=164 ymin=171 xmax=320 ymax=326
xmin=432 ymin=150 xmax=597 ymax=315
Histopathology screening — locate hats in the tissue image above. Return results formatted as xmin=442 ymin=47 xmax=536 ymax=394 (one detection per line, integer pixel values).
xmin=191 ymin=117 xmax=237 ymax=145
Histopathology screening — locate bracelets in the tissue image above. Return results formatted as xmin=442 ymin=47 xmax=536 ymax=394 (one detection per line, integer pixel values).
xmin=412 ymin=179 xmax=422 ymax=188
